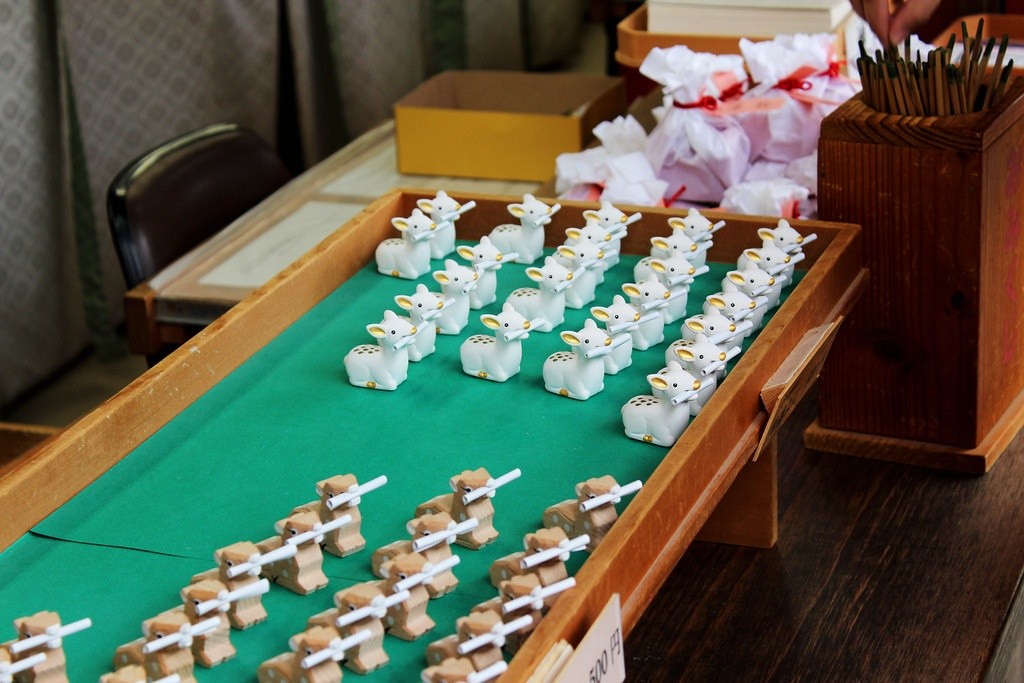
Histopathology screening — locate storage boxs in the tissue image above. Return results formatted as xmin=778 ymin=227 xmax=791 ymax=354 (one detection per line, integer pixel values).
xmin=393 ymin=68 xmax=626 ymax=182
xmin=801 ymin=17 xmax=1022 ymax=477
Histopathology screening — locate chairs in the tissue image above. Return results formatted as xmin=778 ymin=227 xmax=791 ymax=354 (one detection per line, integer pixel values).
xmin=106 ymin=121 xmax=305 ymax=368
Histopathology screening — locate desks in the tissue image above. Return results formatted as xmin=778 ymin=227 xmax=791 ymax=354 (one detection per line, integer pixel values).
xmin=0 ymin=180 xmax=870 ymax=681
xmin=120 ymin=121 xmax=393 ymax=375
